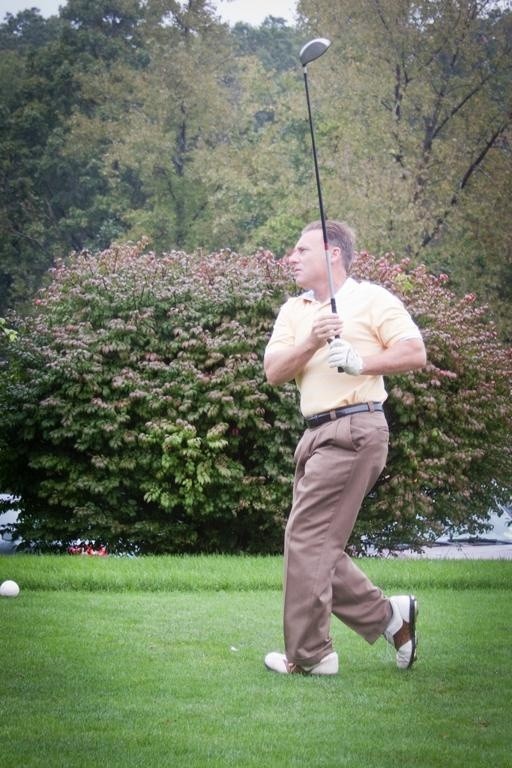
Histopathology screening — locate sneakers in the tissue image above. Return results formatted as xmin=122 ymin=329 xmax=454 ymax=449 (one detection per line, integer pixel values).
xmin=380 ymin=593 xmax=419 ymax=671
xmin=263 ymin=651 xmax=340 ymax=678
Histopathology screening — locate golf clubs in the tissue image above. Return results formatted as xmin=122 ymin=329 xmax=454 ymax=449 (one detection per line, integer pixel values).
xmin=298 ymin=39 xmax=359 ymax=375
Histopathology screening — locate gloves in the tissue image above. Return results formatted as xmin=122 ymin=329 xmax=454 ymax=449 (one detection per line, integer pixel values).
xmin=325 ymin=337 xmax=363 ymax=377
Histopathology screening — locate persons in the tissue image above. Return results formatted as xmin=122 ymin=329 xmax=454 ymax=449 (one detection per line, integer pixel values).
xmin=264 ymin=220 xmax=427 ymax=676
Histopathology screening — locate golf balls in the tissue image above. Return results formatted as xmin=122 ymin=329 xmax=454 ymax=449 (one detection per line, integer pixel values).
xmin=0 ymin=580 xmax=19 ymax=597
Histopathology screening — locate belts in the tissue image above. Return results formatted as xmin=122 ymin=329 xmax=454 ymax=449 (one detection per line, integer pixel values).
xmin=303 ymin=402 xmax=384 ymax=430
